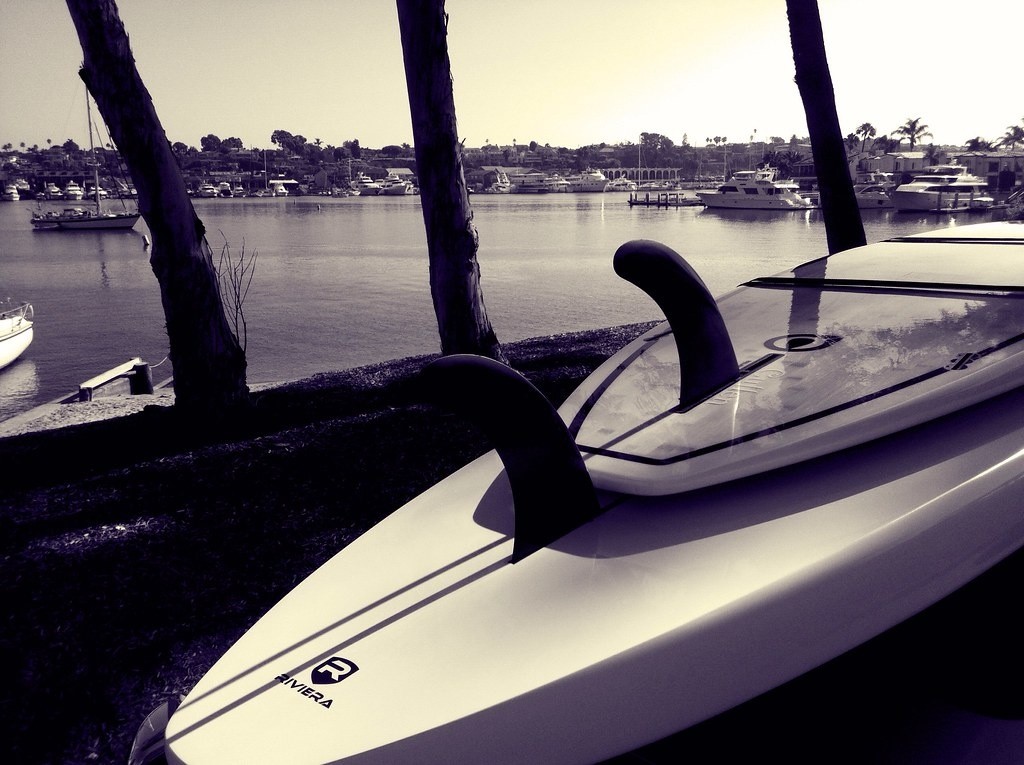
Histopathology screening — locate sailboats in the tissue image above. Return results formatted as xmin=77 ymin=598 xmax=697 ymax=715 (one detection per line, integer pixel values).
xmin=58 ymin=79 xmax=143 ymax=229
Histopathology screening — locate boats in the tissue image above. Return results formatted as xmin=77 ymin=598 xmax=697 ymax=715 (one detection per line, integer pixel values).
xmin=332 ymin=190 xmax=349 ymax=198
xmin=198 ymin=183 xmax=231 ymax=197
xmin=462 ymin=165 xmax=572 ymax=195
xmin=695 ymin=169 xmax=816 ymax=209
xmin=638 ymin=180 xmax=683 ymax=190
xmin=109 ymin=184 xmax=197 ymax=201
xmin=563 ymin=165 xmax=610 ymax=195
xmin=252 ymin=183 xmax=289 ymax=197
xmin=24 ymin=206 xmax=98 ymax=231
xmin=88 ymin=186 xmax=107 ymax=198
xmin=852 ymin=172 xmax=899 ymax=208
xmin=0 ymin=298 xmax=37 ymax=371
xmin=605 ymin=176 xmax=640 ymax=191
xmin=345 ymin=188 xmax=361 ymax=196
xmin=2 ymin=185 xmax=21 ymax=201
xmin=46 ymin=182 xmax=63 ymax=200
xmin=63 ymin=180 xmax=83 ymax=200
xmin=373 ymin=167 xmax=420 ymax=195
xmin=348 ymin=172 xmax=385 ymax=195
xmin=894 ymin=173 xmax=994 ymax=210
xmin=232 ymin=187 xmax=244 ymax=196
